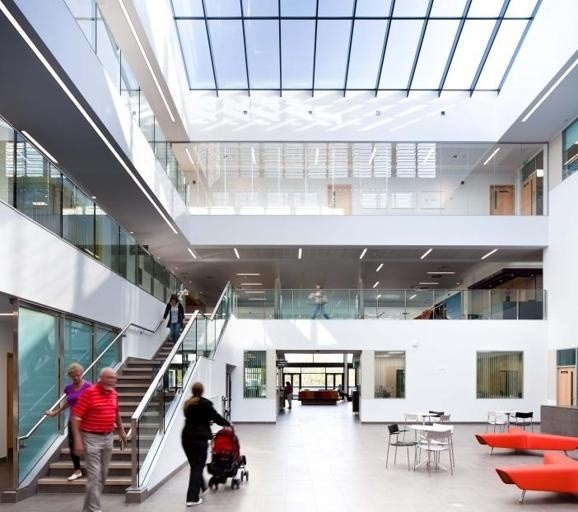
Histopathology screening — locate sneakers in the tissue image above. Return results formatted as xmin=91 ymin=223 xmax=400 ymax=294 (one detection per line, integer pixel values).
xmin=287 ymin=405 xmax=292 ymax=410
xmin=201 ymin=487 xmax=210 ymax=497
xmin=185 ymin=498 xmax=203 ymax=507
xmin=67 ymin=470 xmax=83 ymax=481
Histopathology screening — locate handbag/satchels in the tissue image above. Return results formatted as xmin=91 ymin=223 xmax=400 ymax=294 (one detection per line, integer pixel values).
xmin=312 ymin=293 xmax=329 ymax=305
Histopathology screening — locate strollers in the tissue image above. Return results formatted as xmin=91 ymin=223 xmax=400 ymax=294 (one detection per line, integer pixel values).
xmin=205 ymin=422 xmax=250 ymax=491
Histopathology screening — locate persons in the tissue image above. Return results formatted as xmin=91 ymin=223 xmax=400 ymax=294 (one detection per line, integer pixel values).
xmin=160 ymin=293 xmax=185 ymax=345
xmin=44 ymin=364 xmax=94 ymax=481
xmin=180 ymin=382 xmax=233 ymax=507
xmin=70 ymin=367 xmax=128 ymax=512
xmin=308 ymin=282 xmax=331 ymax=319
xmin=284 ymin=382 xmax=293 ymax=410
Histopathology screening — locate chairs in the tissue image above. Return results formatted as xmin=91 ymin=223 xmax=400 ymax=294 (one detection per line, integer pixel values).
xmin=486 ymin=410 xmax=533 ymax=433
xmin=386 ymin=409 xmax=457 ymax=476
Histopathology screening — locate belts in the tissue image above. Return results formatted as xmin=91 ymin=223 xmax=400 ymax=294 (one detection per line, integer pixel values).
xmin=83 ymin=431 xmax=112 ymax=436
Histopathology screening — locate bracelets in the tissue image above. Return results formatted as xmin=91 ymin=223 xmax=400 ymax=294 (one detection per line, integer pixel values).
xmin=54 ymin=410 xmax=57 ymax=413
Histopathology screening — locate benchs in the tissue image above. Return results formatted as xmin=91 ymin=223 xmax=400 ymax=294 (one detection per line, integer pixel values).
xmin=496 ymin=452 xmax=578 ymax=501
xmin=475 ymin=426 xmax=578 ymax=456
